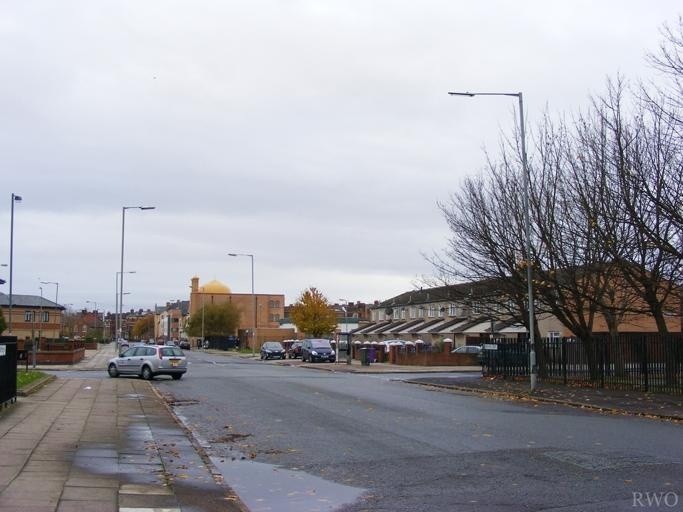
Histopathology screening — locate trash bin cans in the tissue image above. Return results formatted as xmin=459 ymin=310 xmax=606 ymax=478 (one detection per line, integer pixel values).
xmin=360 ymin=348 xmax=369 ymax=365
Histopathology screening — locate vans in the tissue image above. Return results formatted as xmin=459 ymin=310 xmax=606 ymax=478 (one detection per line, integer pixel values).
xmin=301 ymin=338 xmax=336 ymax=363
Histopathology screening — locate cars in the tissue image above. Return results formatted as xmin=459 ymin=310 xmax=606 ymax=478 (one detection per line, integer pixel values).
xmin=287 ymin=342 xmax=303 ymax=359
xmin=106 ymin=338 xmax=190 ymax=380
xmin=260 ymin=342 xmax=287 ymax=360
xmin=451 ymin=346 xmax=482 ymax=353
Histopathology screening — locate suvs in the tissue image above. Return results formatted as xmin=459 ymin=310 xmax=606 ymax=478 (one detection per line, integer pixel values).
xmin=224 ymin=336 xmax=238 ymax=348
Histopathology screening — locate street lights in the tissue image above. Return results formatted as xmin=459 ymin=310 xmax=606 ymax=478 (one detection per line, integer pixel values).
xmin=338 ymin=299 xmax=350 ymax=365
xmin=188 ymin=285 xmax=204 ymax=353
xmin=9 ymin=193 xmax=22 ymax=336
xmin=38 ymin=281 xmax=58 ymax=352
xmin=119 ymin=206 xmax=155 ymax=341
xmin=85 ymin=300 xmax=97 ymax=330
xmin=449 ymin=92 xmax=537 ymax=392
xmin=228 ymin=253 xmax=256 ymax=356
xmin=114 ymin=271 xmax=136 ymax=349
